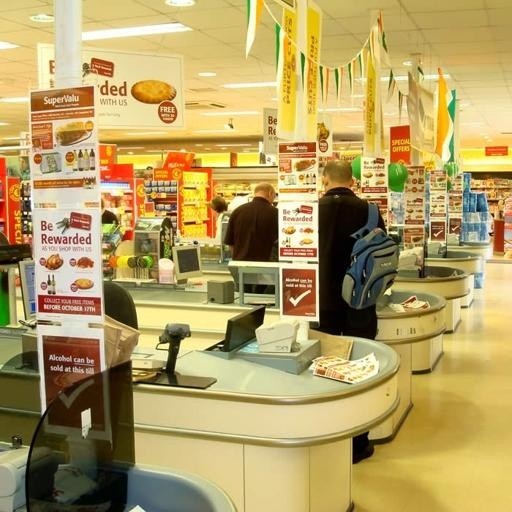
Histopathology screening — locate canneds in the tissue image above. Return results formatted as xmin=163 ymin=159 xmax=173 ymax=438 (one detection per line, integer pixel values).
xmin=70 ymin=212 xmax=91 ymax=230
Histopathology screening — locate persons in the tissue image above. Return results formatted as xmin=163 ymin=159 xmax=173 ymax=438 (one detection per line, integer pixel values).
xmin=211 ymin=198 xmax=228 ymax=239
xmin=320 ymin=161 xmax=387 ymax=340
xmin=224 ymin=183 xmax=278 ymax=293
xmin=101 ymin=199 xmax=118 ymax=223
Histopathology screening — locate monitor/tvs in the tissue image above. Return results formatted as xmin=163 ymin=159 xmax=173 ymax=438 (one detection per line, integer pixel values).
xmin=222 ymin=304 xmax=266 ymax=352
xmin=172 ymin=244 xmax=203 ymax=286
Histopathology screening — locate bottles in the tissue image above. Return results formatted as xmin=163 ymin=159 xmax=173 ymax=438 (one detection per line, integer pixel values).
xmin=303 ymin=173 xmax=316 ymax=185
xmin=72 ymin=149 xmax=96 ymax=170
xmin=47 ymin=274 xmax=56 ymax=294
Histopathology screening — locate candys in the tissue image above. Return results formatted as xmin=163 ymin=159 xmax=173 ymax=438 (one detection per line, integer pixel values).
xmin=109 ymin=256 xmax=153 ymax=281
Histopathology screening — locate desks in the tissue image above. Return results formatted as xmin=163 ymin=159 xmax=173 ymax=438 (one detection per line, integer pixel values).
xmin=1 ymin=217 xmax=496 ymax=510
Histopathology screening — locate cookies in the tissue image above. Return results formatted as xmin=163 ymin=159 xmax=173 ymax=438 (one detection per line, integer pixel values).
xmin=131 ymin=80 xmax=177 ymax=103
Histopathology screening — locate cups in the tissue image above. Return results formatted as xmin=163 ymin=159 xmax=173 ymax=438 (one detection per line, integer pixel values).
xmin=285 ymin=237 xmax=291 ymax=248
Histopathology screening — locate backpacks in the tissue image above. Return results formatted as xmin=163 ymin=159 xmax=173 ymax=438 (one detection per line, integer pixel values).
xmin=342 ymin=201 xmax=399 ymax=309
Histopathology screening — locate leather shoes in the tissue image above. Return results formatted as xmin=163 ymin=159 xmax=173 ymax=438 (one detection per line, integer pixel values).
xmin=353 ymin=443 xmax=375 ymax=462
